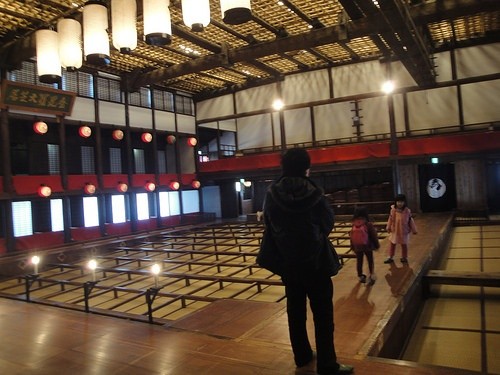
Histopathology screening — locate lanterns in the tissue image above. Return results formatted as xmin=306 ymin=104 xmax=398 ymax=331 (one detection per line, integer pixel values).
xmin=181 ymin=0 xmax=211 ymax=32
xmin=110 ymin=0 xmax=139 ymax=54
xmin=82 ymin=0 xmax=111 ymax=67
xmin=34 ymin=25 xmax=62 ymax=84
xmin=220 ymin=0 xmax=252 ymax=25
xmin=142 ymin=0 xmax=172 ymax=46
xmin=56 ymin=16 xmax=83 ymax=72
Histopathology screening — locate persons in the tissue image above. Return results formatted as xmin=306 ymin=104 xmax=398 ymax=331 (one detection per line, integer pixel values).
xmin=256 ymin=148 xmax=355 ymax=375
xmin=384 ymin=195 xmax=417 ymax=263
xmin=352 ymin=205 xmax=380 ymax=284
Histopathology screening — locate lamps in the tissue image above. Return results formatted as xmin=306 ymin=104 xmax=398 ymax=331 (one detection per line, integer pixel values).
xmin=35 ymin=0 xmax=253 ymax=84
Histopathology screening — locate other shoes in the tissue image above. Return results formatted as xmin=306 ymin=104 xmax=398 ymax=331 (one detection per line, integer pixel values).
xmin=384 ymin=259 xmax=394 ymax=263
xmin=370 ymin=278 xmax=375 ymax=284
xmin=312 ymin=352 xmax=317 ymax=360
xmin=400 ymin=258 xmax=407 ymax=262
xmin=337 ymin=364 xmax=352 ymax=374
xmin=358 ymin=274 xmax=366 ymax=278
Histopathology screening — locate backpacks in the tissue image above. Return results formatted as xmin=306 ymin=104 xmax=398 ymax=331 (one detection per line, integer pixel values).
xmin=351 ymin=220 xmax=368 ymax=246
